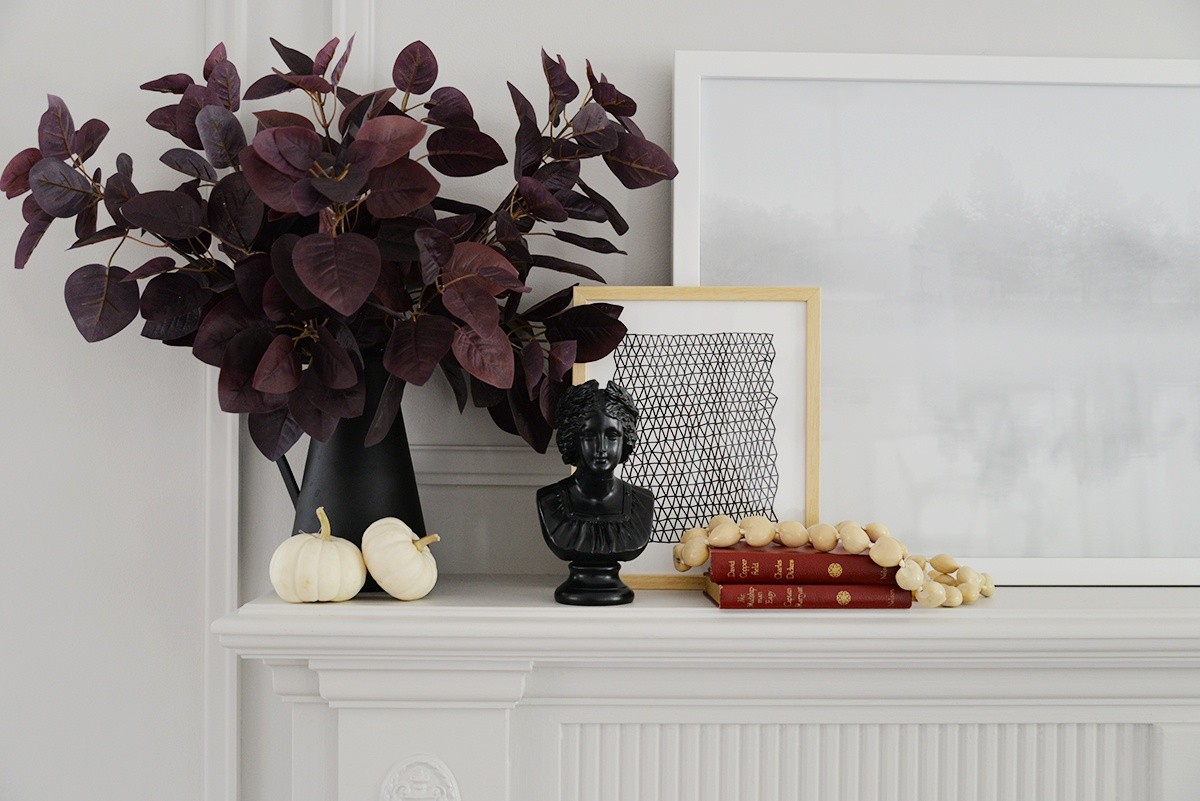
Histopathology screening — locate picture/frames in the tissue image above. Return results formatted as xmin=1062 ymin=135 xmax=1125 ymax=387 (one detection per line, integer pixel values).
xmin=673 ymin=49 xmax=1200 ymax=589
xmin=570 ymin=286 xmax=822 ymax=590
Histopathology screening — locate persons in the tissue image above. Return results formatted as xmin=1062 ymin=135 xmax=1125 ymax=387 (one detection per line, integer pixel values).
xmin=536 ymin=381 xmax=654 ymax=563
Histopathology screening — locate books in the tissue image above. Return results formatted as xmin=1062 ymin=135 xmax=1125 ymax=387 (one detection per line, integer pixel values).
xmin=704 ymin=545 xmax=915 ymax=608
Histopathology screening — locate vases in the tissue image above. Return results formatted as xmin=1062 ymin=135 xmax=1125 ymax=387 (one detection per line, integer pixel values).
xmin=277 ymin=346 xmax=430 ymax=601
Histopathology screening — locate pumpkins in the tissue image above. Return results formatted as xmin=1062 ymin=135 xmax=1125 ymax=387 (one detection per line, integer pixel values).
xmin=362 ymin=516 xmax=439 ymax=600
xmin=270 ymin=506 xmax=367 ymax=602
